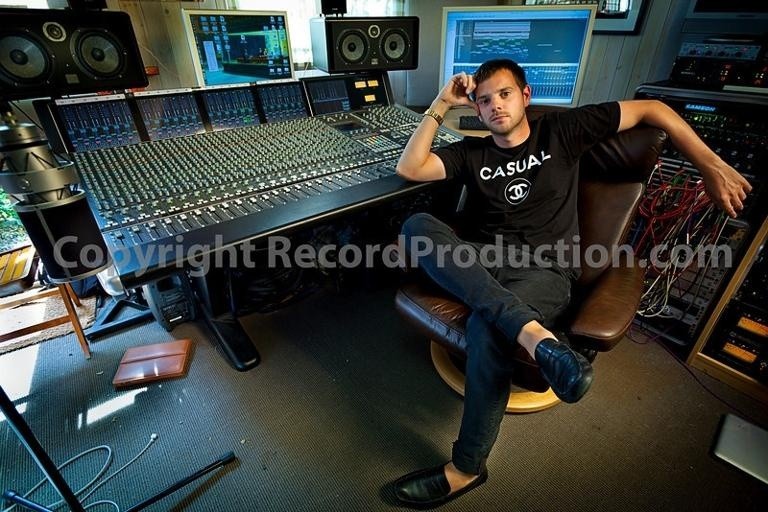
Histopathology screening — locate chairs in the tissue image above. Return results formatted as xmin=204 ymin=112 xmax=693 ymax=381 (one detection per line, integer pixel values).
xmin=394 ymin=104 xmax=669 ymax=414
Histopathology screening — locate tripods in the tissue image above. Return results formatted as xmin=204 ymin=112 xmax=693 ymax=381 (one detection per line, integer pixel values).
xmin=0 ymin=385 xmax=236 ymax=512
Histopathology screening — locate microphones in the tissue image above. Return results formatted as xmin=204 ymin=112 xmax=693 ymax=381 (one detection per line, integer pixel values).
xmin=0 ymin=103 xmax=114 ymax=285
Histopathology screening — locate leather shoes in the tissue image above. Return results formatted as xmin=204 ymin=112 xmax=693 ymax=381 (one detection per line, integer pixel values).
xmin=535 ymin=338 xmax=595 ymax=404
xmin=393 ymin=459 xmax=487 ymax=509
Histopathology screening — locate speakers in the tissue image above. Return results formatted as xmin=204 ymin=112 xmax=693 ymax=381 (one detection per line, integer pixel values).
xmin=0 ymin=8 xmax=149 ymax=103
xmin=311 ymin=15 xmax=420 ymax=74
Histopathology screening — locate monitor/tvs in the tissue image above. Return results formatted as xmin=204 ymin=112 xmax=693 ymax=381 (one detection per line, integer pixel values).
xmin=438 ymin=3 xmax=598 ymax=109
xmin=181 ymin=7 xmax=296 ymax=88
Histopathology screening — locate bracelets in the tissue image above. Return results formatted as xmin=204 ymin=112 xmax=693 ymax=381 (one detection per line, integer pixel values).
xmin=424 ymin=109 xmax=443 ymax=125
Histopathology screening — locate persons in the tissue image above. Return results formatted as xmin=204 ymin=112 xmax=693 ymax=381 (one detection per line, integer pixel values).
xmin=394 ymin=54 xmax=753 ymax=510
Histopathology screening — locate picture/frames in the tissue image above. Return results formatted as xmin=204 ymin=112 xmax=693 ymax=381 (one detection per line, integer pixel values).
xmin=522 ymin=0 xmax=650 ymax=36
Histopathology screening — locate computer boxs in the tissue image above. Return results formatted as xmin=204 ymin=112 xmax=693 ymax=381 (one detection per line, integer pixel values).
xmin=141 ymin=271 xmax=196 ymax=333
